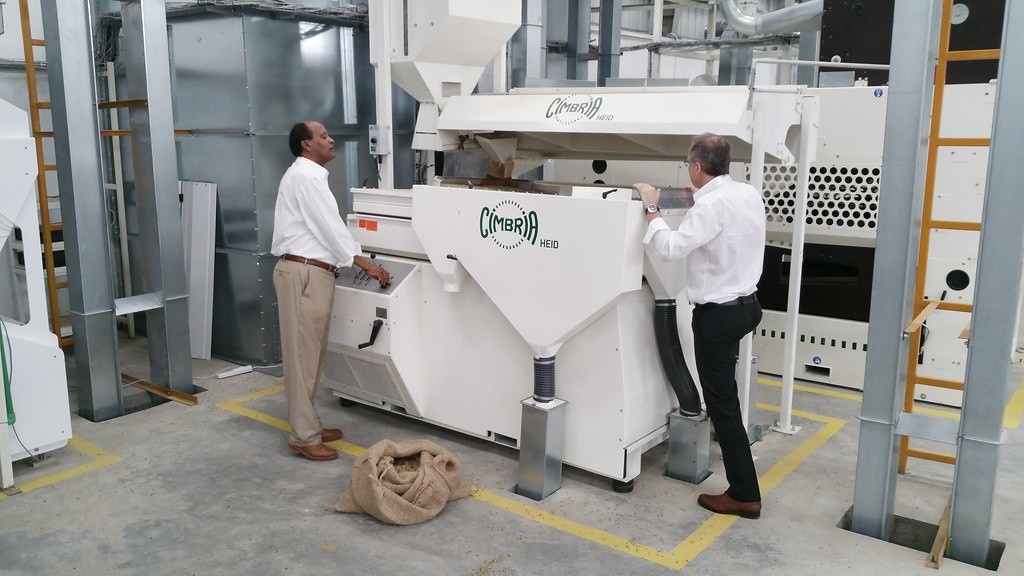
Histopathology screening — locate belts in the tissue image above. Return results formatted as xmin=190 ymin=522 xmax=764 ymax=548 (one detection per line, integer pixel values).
xmin=696 ymin=292 xmax=757 ymax=308
xmin=282 ymin=254 xmax=338 ymax=274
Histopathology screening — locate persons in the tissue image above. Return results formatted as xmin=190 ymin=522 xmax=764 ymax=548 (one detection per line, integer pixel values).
xmin=270 ymin=120 xmax=390 ymax=461
xmin=633 ymin=133 xmax=766 ymax=518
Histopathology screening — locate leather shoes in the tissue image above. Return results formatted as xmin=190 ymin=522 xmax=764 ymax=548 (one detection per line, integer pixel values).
xmin=698 ymin=490 xmax=762 ymax=520
xmin=320 ymin=425 xmax=343 ymax=442
xmin=289 ymin=441 xmax=337 ymax=461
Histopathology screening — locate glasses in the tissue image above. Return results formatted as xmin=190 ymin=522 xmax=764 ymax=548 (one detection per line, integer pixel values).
xmin=684 ymin=160 xmax=704 ymax=168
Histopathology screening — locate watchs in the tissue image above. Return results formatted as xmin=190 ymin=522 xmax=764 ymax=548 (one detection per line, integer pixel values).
xmin=644 ymin=204 xmax=659 ymax=215
